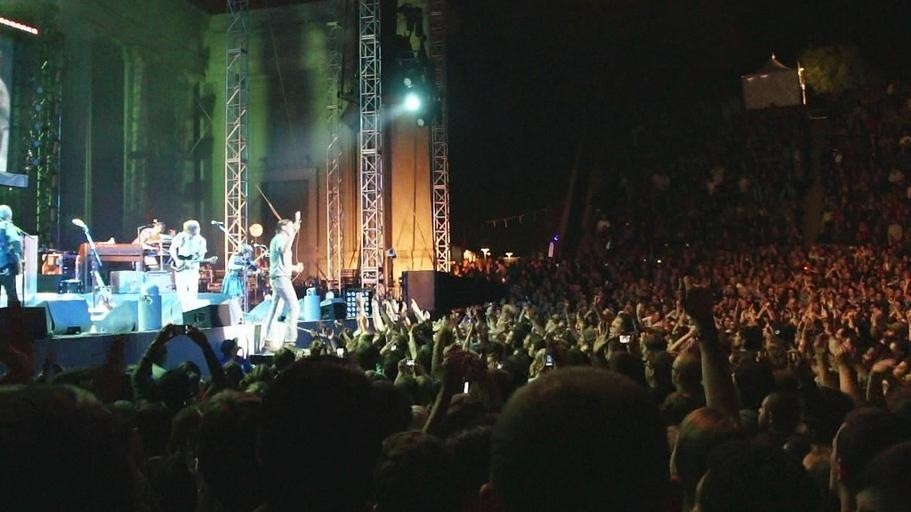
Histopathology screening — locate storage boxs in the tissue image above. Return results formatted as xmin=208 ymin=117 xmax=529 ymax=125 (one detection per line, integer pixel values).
xmin=57 ymin=280 xmax=82 ymax=296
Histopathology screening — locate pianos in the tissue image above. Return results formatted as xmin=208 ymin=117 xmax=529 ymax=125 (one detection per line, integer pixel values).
xmin=79 ymin=244 xmax=142 ymax=293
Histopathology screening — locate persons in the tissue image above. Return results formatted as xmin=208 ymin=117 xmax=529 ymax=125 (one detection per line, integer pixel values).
xmin=1 ymin=289 xmax=911 ymax=510
xmin=132 ymin=222 xmax=165 ymax=268
xmin=220 ymin=244 xmax=262 ymax=296
xmin=263 ymin=211 xmax=304 ymax=351
xmin=454 ymin=80 xmax=911 ymax=289
xmin=169 ymin=219 xmax=207 ymax=306
xmin=0 ymin=204 xmax=24 ymax=307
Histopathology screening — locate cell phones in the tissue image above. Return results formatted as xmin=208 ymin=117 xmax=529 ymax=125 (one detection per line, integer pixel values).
xmin=463 ymin=380 xmax=469 ymax=394
xmin=545 ymin=354 xmax=553 ymax=366
xmin=335 ymin=347 xmax=345 ymax=357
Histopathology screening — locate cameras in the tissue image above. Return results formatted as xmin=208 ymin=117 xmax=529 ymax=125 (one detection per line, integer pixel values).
xmin=618 ymin=335 xmax=631 ymax=344
xmin=405 ymin=359 xmax=415 ymax=366
xmin=172 ymin=325 xmax=191 ymax=335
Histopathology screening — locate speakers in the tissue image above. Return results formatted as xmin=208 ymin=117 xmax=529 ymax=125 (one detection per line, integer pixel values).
xmin=36 ymin=299 xmax=91 ymax=334
xmin=400 ymin=269 xmax=445 ymax=314
xmin=182 ymin=304 xmax=231 ymax=326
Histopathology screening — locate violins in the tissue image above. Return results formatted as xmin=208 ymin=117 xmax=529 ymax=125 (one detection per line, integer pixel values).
xmin=241 ymin=256 xmax=266 ymax=278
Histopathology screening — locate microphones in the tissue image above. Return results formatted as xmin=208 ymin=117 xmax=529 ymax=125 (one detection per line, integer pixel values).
xmin=71 ymin=218 xmax=89 ymax=233
xmin=210 ymin=219 xmax=223 ymax=225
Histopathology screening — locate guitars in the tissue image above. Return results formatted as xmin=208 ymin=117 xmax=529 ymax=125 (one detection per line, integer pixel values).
xmin=170 ymin=255 xmax=218 ymax=271
